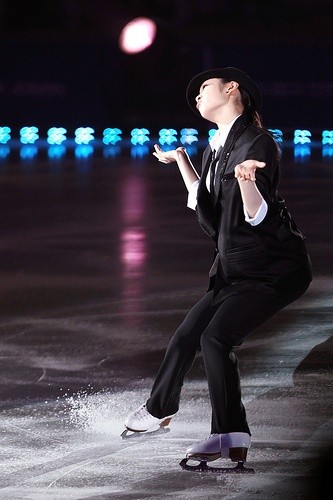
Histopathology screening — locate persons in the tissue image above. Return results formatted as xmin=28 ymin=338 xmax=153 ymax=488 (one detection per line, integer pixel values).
xmin=120 ymin=66 xmax=314 ymax=473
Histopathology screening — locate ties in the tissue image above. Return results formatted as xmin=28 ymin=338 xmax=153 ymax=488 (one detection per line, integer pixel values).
xmin=210 ymin=146 xmax=223 ymax=196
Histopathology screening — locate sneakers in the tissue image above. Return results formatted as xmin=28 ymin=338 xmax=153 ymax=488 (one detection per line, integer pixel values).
xmin=120 ymin=402 xmax=179 ymax=440
xmin=179 ymin=432 xmax=254 ymax=473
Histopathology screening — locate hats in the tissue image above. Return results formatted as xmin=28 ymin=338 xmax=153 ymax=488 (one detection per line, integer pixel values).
xmin=186 ymin=67 xmax=262 ymax=121
